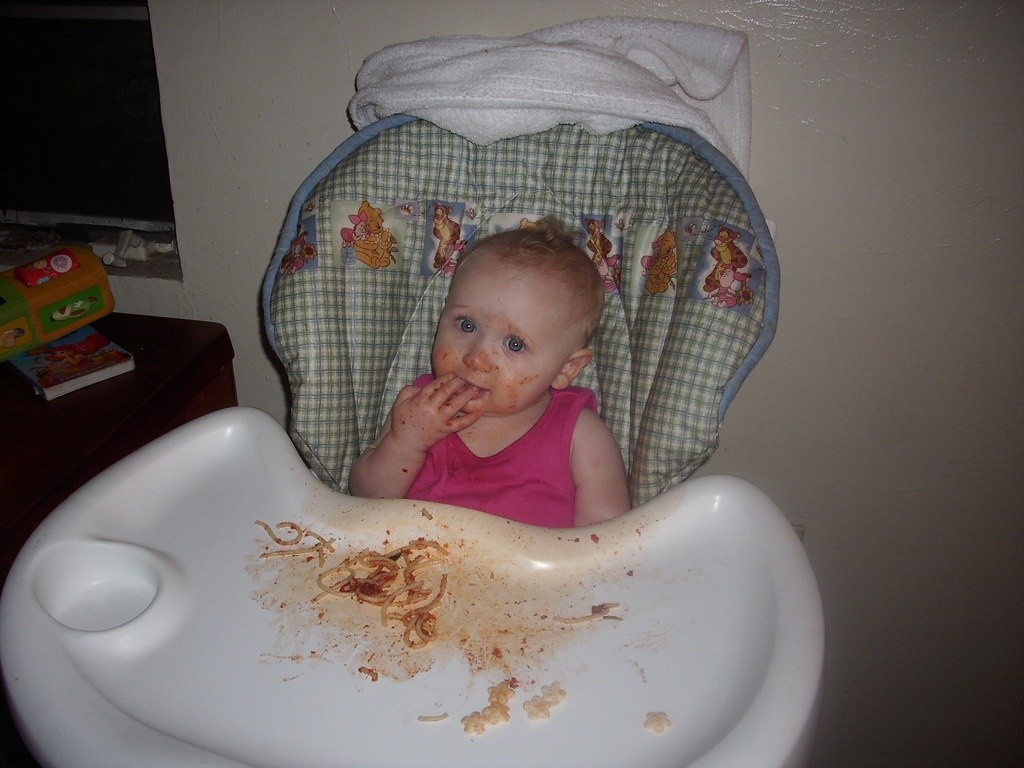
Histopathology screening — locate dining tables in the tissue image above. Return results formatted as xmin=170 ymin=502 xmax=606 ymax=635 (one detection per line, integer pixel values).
xmin=3 ymin=404 xmax=834 ymax=767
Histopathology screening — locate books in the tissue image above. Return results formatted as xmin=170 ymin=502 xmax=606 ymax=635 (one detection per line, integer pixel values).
xmin=6 ymin=323 xmax=135 ymax=402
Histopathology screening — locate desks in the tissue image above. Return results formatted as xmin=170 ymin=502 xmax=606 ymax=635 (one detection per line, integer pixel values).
xmin=0 ymin=312 xmax=240 ymax=597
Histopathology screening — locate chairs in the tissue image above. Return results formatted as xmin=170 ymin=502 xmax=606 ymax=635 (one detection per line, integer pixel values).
xmin=259 ymin=104 xmax=786 ymax=525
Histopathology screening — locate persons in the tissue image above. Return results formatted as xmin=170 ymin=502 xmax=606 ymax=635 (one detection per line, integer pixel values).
xmin=347 ymin=217 xmax=633 ymax=527
